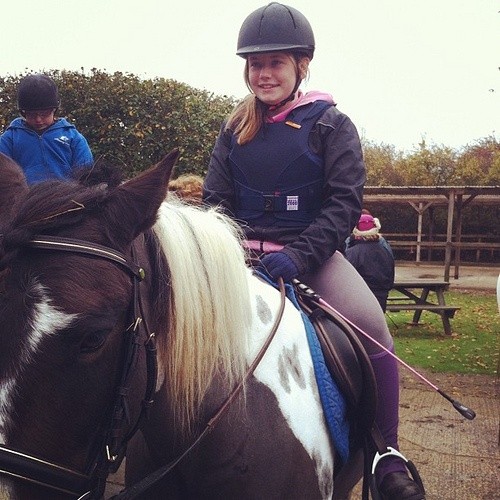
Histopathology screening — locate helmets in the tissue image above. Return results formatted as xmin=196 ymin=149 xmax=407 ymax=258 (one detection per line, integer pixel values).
xmin=16 ymin=74 xmax=58 ymax=110
xmin=236 ymin=2 xmax=315 ymax=58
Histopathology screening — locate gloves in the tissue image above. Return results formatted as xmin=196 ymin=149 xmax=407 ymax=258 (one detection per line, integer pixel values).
xmin=256 ymin=252 xmax=299 ymax=285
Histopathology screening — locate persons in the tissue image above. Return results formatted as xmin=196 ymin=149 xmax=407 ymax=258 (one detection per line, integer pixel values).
xmin=201 ymin=3 xmax=423 ymax=500
xmin=345 ymin=214 xmax=396 ymax=313
xmin=0 ymin=75 xmax=94 ymax=188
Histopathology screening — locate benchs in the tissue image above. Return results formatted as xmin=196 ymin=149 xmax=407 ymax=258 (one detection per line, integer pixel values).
xmin=386 ymin=296 xmax=462 ymax=312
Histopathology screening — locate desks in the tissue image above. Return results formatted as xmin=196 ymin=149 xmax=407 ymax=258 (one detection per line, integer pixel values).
xmin=392 ymin=280 xmax=452 ymax=333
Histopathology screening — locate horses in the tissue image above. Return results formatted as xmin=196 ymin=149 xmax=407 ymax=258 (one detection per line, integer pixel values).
xmin=0 ymin=146 xmax=381 ymax=500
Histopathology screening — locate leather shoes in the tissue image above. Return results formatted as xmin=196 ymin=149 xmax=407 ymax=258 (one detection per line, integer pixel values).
xmin=379 ymin=470 xmax=424 ymax=500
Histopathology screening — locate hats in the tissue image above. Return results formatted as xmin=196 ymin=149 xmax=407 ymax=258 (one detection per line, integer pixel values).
xmin=352 ymin=214 xmax=381 ymax=236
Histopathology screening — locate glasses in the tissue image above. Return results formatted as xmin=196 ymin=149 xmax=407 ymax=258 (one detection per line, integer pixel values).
xmin=25 ymin=110 xmax=53 ymax=119
